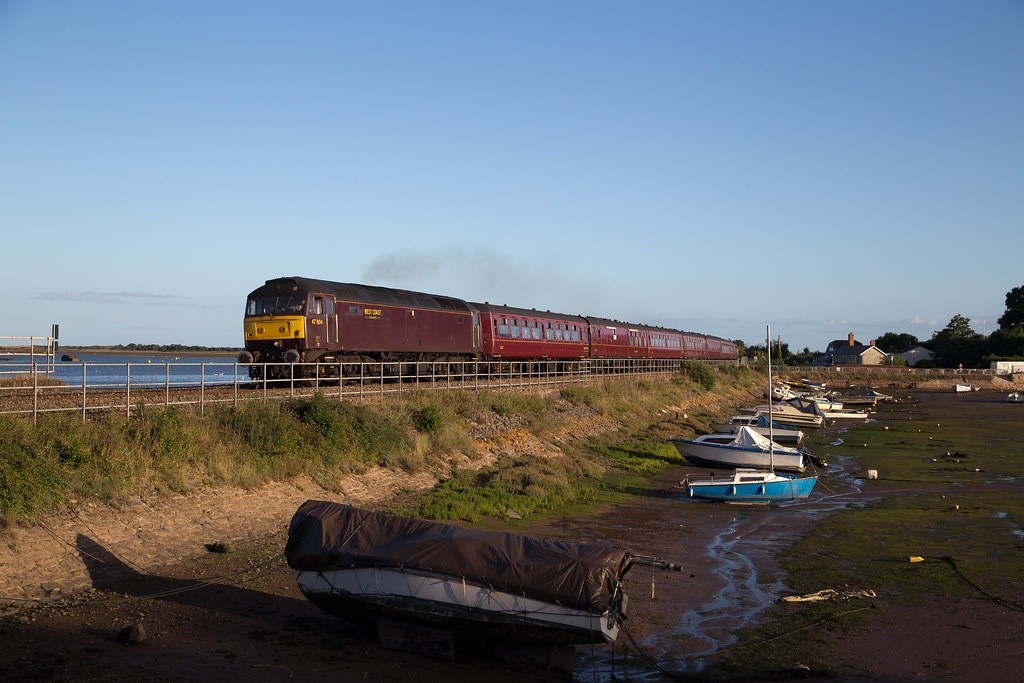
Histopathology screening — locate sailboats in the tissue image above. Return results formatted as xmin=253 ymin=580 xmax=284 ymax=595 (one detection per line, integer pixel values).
xmin=679 ymin=323 xmax=820 ymax=502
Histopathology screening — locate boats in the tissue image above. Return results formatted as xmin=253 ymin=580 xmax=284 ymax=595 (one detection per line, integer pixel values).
xmin=1006 ymin=391 xmax=1024 ymax=402
xmin=738 ymin=401 xmax=838 ymax=428
xmin=282 ymin=499 xmax=684 ymax=648
xmin=669 ymin=424 xmax=829 ymax=474
xmin=951 ymin=384 xmax=972 ymax=393
xmin=765 ymin=377 xmax=893 ymax=420
xmin=708 ymin=414 xmax=803 ymax=445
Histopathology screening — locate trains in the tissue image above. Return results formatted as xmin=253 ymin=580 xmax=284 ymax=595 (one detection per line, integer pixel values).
xmin=237 ymin=277 xmax=741 ymax=387
